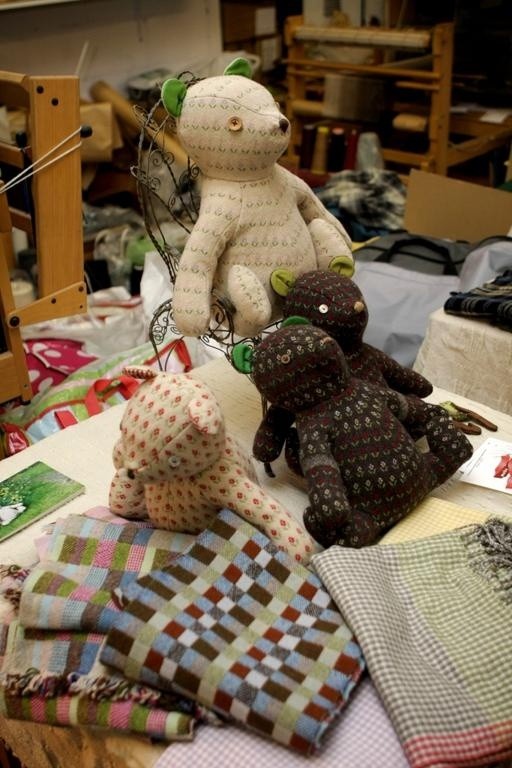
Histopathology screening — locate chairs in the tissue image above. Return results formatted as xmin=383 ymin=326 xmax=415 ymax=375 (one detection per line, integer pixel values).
xmin=353 ymin=168 xmax=512 ymax=253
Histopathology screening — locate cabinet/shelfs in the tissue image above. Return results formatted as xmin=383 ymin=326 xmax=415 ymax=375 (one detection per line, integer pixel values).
xmin=284 ymin=14 xmax=512 ymax=176
xmin=0 ymin=69 xmax=88 ymax=413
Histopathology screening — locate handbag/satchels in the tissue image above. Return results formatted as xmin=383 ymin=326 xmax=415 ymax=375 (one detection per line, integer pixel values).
xmin=349 ymin=226 xmax=512 ymax=277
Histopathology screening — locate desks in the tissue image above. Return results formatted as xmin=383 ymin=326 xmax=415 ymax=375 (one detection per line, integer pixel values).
xmin=0 ymin=346 xmax=512 ymax=536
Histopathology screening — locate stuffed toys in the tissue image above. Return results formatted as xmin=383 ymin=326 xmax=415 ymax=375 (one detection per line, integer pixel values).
xmin=161 ymin=57 xmax=354 ymax=339
xmin=269 ymin=255 xmax=452 ymax=481
xmin=231 ymin=313 xmax=475 ymax=550
xmin=107 ymin=364 xmax=315 ymax=566
